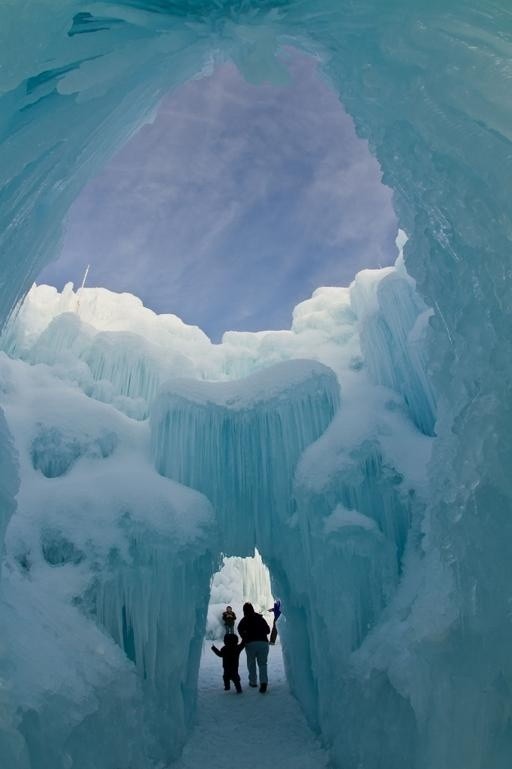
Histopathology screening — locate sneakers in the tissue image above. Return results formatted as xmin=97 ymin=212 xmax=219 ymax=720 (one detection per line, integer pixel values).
xmin=236 ymin=687 xmax=241 ymax=692
xmin=249 ymin=682 xmax=257 ymax=687
xmin=269 ymin=642 xmax=274 ymax=645
xmin=224 ymin=688 xmax=229 ymax=690
xmin=259 ymin=684 xmax=266 ymax=692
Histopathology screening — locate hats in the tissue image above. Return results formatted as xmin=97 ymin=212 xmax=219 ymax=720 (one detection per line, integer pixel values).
xmin=224 ymin=634 xmax=238 ymax=645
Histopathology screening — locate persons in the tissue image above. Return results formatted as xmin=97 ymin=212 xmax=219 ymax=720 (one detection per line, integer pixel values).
xmin=210 ymin=634 xmax=246 ymax=694
xmin=222 ymin=605 xmax=237 ymax=635
xmin=266 ymin=602 xmax=282 ymax=645
xmin=237 ymin=602 xmax=271 ymax=694
